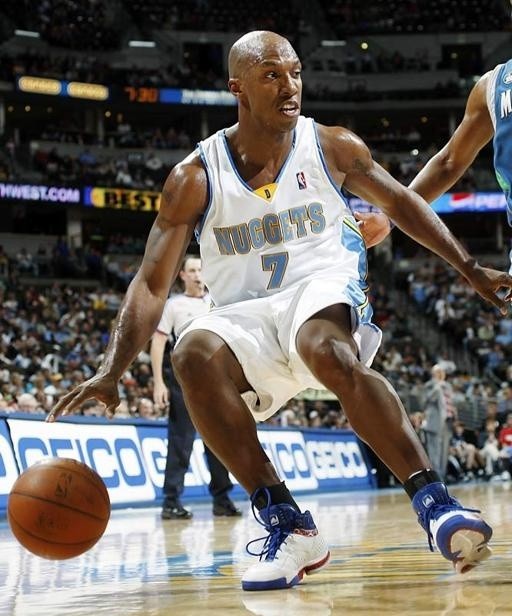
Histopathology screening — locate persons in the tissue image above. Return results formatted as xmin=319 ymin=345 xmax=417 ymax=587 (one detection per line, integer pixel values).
xmin=45 ymin=29 xmax=512 ymax=591
xmin=1 ymin=0 xmax=512 ymax=521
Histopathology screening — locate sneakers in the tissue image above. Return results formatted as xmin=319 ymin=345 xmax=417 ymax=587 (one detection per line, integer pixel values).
xmin=411 ymin=481 xmax=494 ymax=575
xmin=213 ymin=499 xmax=244 ymax=517
xmin=161 ymin=503 xmax=192 ymax=520
xmin=242 ymin=503 xmax=331 ymax=591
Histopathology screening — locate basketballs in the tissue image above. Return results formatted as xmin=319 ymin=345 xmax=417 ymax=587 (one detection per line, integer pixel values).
xmin=8 ymin=457 xmax=109 ymax=560
